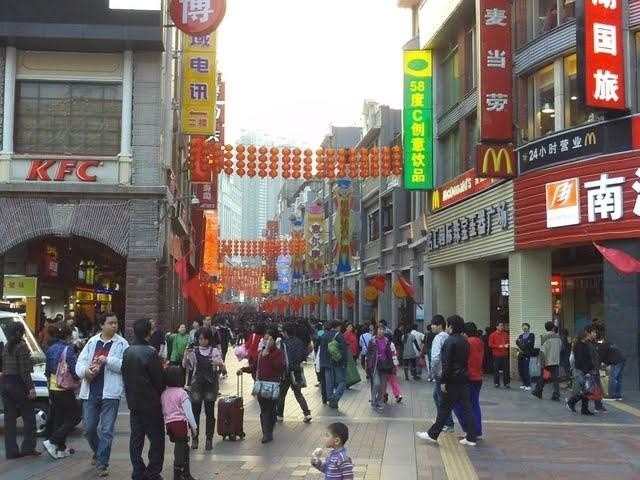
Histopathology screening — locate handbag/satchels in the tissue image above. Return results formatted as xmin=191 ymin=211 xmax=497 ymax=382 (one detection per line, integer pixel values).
xmin=252 ymin=380 xmax=281 ymax=401
xmin=56 ymin=362 xmax=80 ymax=390
xmin=175 ymin=354 xmax=183 ymax=364
xmin=235 ymin=345 xmax=249 ymax=361
xmin=422 ymin=343 xmax=428 ymax=354
xmin=290 ymin=369 xmax=307 ymax=391
xmin=418 ymin=356 xmax=426 ymax=368
xmin=328 ymin=340 xmax=342 ymax=362
xmin=377 ymin=358 xmax=395 ymax=374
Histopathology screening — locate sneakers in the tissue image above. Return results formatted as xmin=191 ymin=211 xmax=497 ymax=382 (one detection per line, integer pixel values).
xmin=206 ymin=440 xmax=212 ymax=450
xmin=566 ymin=397 xmax=623 ymax=416
xmin=495 ymin=384 xmax=510 ymax=388
xmin=458 ymin=434 xmax=483 ymax=446
xmin=262 ymin=432 xmax=273 ymax=443
xmin=191 ymin=440 xmax=198 ymax=449
xmin=371 ymin=394 xmax=403 ymax=411
xmin=303 ymin=415 xmax=312 ymax=422
xmin=433 ymin=420 xmax=455 ymax=433
xmin=22 ymin=448 xmax=42 ymax=455
xmin=91 ymin=455 xmax=109 ymax=477
xmin=405 ymin=376 xmax=436 ymax=382
xmin=42 ymin=439 xmax=70 ymax=460
xmin=520 ymin=385 xmax=560 ymax=402
xmin=7 ymin=452 xmax=24 ymax=459
xmin=416 ymin=431 xmax=437 ymax=443
xmin=277 ymin=416 xmax=283 ymax=422
xmin=329 ymin=399 xmax=338 ymax=409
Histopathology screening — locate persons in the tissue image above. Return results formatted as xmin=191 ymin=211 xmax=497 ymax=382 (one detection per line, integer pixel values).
xmin=311 ymin=423 xmax=354 ymax=480
xmin=0 ymin=310 xmax=87 ymax=459
xmin=75 ymin=311 xmax=197 ymax=480
xmin=491 ymin=321 xmax=624 ymax=415
xmin=310 ymin=315 xmax=489 ymax=409
xmin=182 ymin=311 xmax=312 ymax=450
xmin=415 ymin=315 xmax=485 ymax=445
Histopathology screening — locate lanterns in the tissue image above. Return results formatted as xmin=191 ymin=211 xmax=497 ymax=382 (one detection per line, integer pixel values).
xmin=184 ymin=136 xmax=402 ymax=180
xmin=218 ymin=236 xmax=307 ymax=258
xmin=218 ymin=264 xmax=261 ymax=291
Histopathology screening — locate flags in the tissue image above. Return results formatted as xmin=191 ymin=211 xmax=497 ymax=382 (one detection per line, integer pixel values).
xmin=373 ymin=277 xmax=387 ymax=293
xmin=183 ymin=271 xmax=219 ymax=316
xmin=400 ymin=278 xmax=413 ymax=298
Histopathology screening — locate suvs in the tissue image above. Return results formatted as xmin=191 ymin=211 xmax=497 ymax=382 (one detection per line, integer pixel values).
xmin=0 ymin=311 xmax=83 ymax=437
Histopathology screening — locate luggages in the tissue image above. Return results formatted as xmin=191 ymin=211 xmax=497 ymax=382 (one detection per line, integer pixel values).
xmin=216 ymin=371 xmax=245 ymax=441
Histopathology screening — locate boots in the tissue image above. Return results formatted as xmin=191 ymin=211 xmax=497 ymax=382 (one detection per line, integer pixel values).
xmin=174 ymin=461 xmax=195 ymax=480
